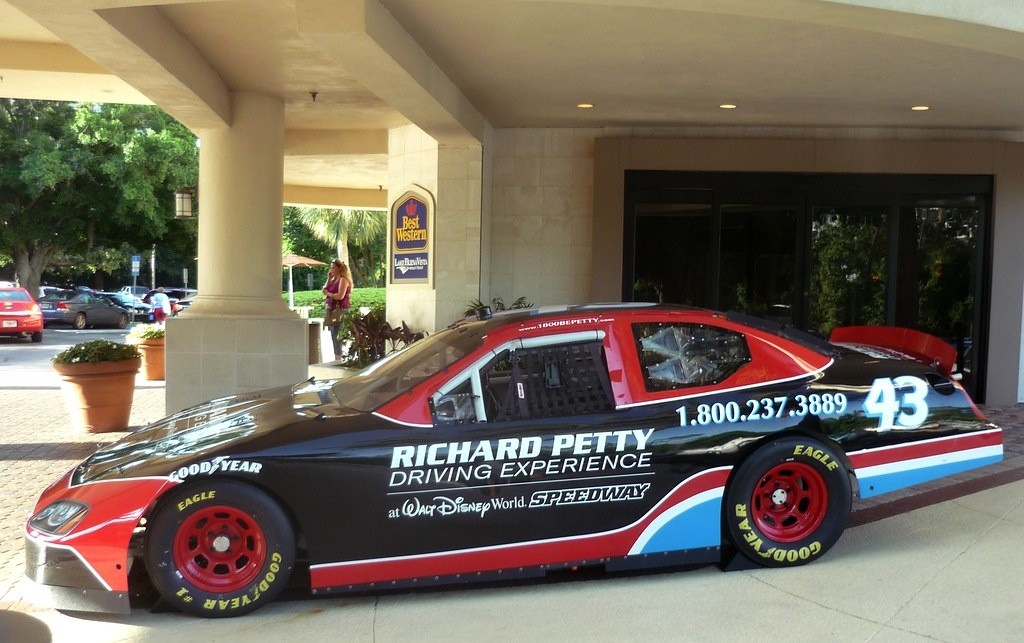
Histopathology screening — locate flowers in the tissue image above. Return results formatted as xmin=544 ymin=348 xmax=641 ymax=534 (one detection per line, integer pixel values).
xmin=50 ymin=340 xmax=143 ymax=364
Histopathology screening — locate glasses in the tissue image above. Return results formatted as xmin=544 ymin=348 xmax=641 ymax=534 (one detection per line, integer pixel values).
xmin=331 ymin=260 xmax=338 ymax=267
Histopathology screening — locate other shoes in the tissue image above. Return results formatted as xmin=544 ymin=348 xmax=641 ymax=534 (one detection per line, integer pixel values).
xmin=328 ymin=360 xmax=342 ymax=366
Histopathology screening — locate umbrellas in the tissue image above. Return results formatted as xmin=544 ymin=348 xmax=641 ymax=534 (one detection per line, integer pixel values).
xmin=282 ymin=254 xmax=329 ymax=308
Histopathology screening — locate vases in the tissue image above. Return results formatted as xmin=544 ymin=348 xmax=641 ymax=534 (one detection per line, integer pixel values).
xmin=52 ymin=357 xmax=141 ymax=433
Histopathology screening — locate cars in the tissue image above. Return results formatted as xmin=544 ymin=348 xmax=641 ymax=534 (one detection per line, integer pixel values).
xmin=25 ymin=300 xmax=1004 ymax=619
xmin=96 ymin=291 xmax=153 ymax=324
xmin=38 ymin=289 xmax=131 ymax=331
xmin=120 ymin=286 xmax=198 ymax=317
xmin=1 ymin=280 xmax=91 ymax=300
xmin=0 ymin=286 xmax=44 ymax=343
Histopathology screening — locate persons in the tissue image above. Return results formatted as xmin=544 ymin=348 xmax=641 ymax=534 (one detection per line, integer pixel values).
xmin=151 ymin=288 xmax=169 ymax=326
xmin=172 ymin=302 xmax=179 ymax=317
xmin=322 ymin=259 xmax=351 ymax=363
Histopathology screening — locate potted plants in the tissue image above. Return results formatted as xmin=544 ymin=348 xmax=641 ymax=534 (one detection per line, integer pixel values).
xmin=136 ymin=328 xmax=165 ymax=380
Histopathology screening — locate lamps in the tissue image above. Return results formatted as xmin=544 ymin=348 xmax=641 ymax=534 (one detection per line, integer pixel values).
xmin=173 ymin=186 xmax=198 ymax=220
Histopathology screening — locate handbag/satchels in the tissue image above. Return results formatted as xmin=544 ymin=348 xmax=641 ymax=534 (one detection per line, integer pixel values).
xmin=323 ymin=297 xmax=346 ymax=326
xmin=150 ymin=315 xmax=154 ymax=320
